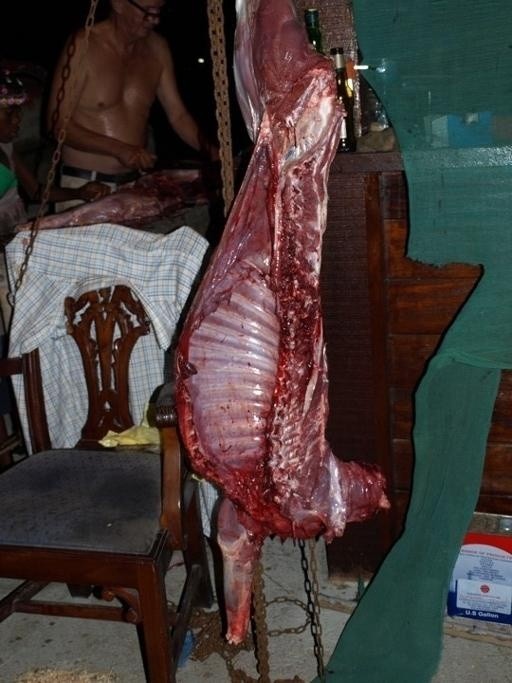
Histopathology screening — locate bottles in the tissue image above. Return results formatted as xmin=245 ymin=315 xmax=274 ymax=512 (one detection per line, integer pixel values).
xmin=328 ymin=48 xmax=356 ymax=153
xmin=305 ymin=6 xmax=324 ymax=54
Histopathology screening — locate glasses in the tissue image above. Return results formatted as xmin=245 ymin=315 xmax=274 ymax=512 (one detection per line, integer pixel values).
xmin=130 ymin=0 xmax=164 ymax=22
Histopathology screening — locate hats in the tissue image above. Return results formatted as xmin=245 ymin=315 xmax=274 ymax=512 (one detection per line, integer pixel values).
xmin=0 ymin=70 xmax=33 ymax=107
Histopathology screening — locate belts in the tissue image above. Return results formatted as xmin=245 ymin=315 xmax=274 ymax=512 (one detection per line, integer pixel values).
xmin=61 ymin=163 xmax=141 ymax=186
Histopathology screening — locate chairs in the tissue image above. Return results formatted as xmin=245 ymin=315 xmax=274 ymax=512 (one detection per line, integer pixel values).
xmin=0 ymin=221 xmax=217 ymax=683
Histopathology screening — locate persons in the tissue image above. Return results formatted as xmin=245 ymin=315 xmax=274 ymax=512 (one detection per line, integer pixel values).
xmin=1 ymin=48 xmax=112 ymax=231
xmin=45 ymin=2 xmax=221 ymax=213
